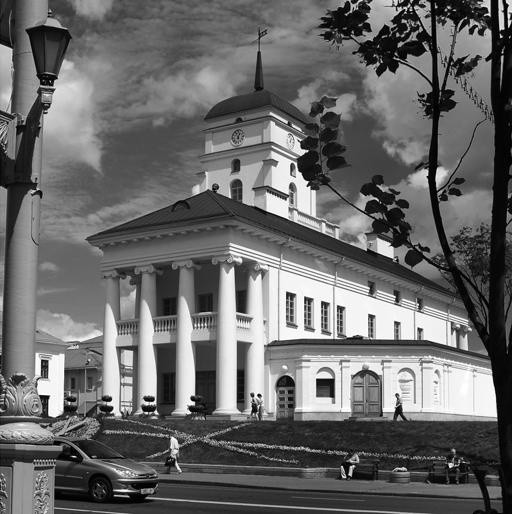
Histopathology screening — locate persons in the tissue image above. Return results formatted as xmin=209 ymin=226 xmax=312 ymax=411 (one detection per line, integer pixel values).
xmin=255 ymin=394 xmax=264 ymax=421
xmin=249 ymin=393 xmax=258 ymax=419
xmin=445 ymin=448 xmax=461 ymax=484
xmin=393 ymin=393 xmax=408 ymax=422
xmin=340 ymin=448 xmax=359 ymax=481
xmin=164 ymin=433 xmax=183 ymax=475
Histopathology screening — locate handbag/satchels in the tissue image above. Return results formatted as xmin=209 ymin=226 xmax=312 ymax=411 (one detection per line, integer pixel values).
xmin=165 ymin=456 xmax=175 ymax=467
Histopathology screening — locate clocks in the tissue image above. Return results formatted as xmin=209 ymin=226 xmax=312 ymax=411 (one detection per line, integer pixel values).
xmin=287 ymin=133 xmax=295 ymax=150
xmin=230 ymin=128 xmax=246 ymax=146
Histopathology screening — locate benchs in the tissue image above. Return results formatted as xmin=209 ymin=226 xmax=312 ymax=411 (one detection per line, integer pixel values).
xmin=338 ymin=458 xmax=380 ymax=480
xmin=428 ymin=460 xmax=468 ymax=484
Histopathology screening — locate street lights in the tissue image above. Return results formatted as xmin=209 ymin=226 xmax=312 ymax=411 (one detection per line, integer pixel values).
xmin=0 ymin=0 xmax=74 ymax=514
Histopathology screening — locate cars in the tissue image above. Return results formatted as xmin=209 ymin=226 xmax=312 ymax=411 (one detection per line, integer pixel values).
xmin=52 ymin=435 xmax=161 ymax=503
